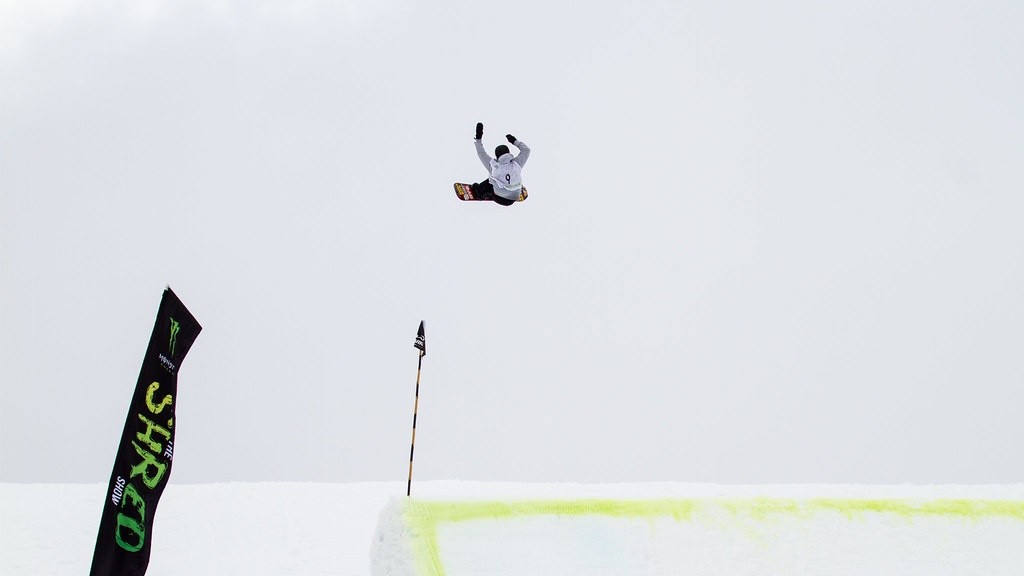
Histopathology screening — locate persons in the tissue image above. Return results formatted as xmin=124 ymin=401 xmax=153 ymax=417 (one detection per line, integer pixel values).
xmin=472 ymin=123 xmax=530 ymax=206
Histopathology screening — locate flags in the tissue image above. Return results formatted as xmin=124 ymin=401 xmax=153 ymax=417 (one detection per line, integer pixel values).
xmin=89 ymin=286 xmax=202 ymax=576
xmin=414 ymin=321 xmax=426 ymax=357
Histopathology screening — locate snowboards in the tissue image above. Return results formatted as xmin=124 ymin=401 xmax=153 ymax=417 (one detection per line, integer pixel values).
xmin=454 ymin=183 xmax=528 ymax=202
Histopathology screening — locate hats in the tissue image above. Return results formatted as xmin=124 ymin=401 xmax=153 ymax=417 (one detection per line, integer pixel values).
xmin=495 ymin=145 xmax=510 ymax=159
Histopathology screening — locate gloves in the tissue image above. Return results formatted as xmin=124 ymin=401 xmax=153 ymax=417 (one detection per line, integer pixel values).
xmin=506 ymin=134 xmax=517 ymax=144
xmin=475 ymin=122 xmax=483 ymax=139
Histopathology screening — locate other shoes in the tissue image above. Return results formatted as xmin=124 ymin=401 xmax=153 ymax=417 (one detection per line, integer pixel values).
xmin=471 ymin=183 xmax=479 ymax=195
